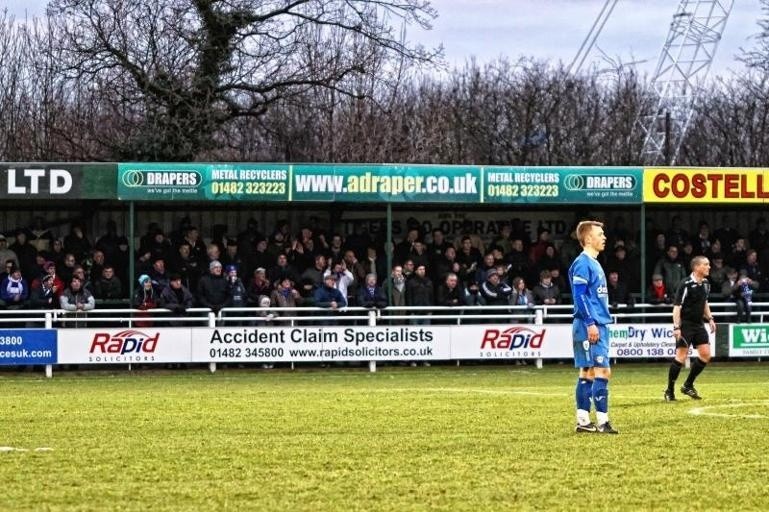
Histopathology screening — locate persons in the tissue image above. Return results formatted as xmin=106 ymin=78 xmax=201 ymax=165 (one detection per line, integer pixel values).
xmin=567 ymin=220 xmax=618 ymax=435
xmin=664 ymin=255 xmax=718 ymax=404
xmin=2 ymin=212 xmax=769 ymax=369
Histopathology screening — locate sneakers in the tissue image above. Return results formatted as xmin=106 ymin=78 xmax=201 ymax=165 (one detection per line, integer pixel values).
xmin=576 ymin=423 xmax=619 ymax=433
xmin=680 ymin=384 xmax=702 ymax=399
xmin=665 ymin=390 xmax=675 ymax=401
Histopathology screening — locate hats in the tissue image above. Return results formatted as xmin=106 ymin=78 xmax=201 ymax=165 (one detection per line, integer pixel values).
xmin=138 ymin=274 xmax=150 ymax=287
xmin=226 ymin=265 xmax=236 ymax=272
